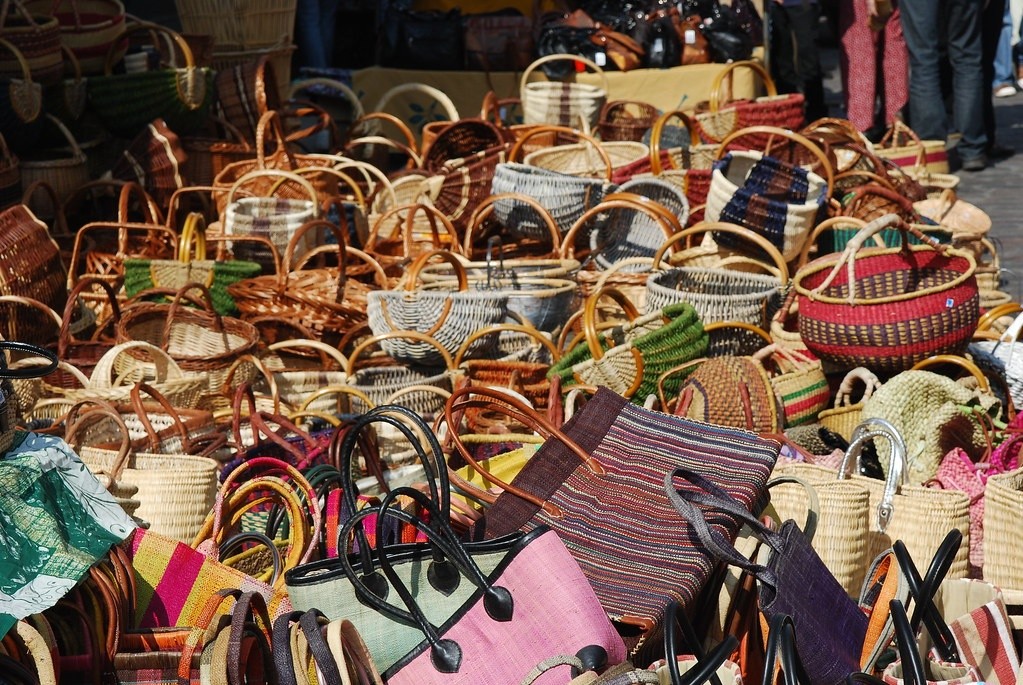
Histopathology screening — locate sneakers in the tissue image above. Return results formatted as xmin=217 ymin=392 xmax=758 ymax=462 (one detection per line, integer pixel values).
xmin=992 ymin=84 xmax=1017 ymax=97
xmin=1015 ymin=63 xmax=1023 ymax=89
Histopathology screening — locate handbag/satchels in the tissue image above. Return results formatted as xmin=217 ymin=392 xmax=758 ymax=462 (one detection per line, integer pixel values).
xmin=0 ymin=380 xmax=1023 ymax=685
xmin=536 ymin=0 xmax=764 ymax=81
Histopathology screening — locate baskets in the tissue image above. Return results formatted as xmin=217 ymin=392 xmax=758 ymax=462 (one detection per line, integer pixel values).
xmin=0 ymin=63 xmax=1013 ymax=548
xmin=0 ymin=0 xmax=297 ymax=135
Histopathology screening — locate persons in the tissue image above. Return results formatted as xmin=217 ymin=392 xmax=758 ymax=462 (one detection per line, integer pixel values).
xmin=298 ymin=0 xmax=334 ymax=69
xmin=993 ymin=0 xmax=1023 ymax=96
xmin=839 ymin=0 xmax=910 ymax=132
xmin=765 ymin=0 xmax=829 ymax=123
xmin=897 ymin=0 xmax=1005 ymax=171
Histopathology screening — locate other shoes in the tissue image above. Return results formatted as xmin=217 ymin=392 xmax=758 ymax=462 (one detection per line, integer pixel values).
xmin=950 ymin=151 xmax=988 ymax=170
